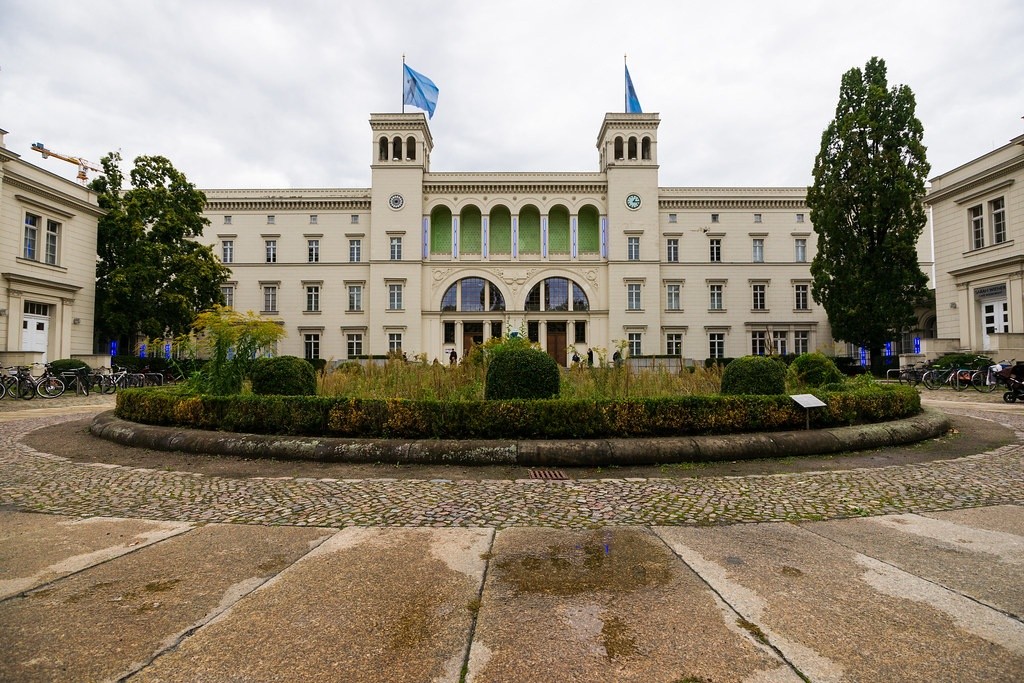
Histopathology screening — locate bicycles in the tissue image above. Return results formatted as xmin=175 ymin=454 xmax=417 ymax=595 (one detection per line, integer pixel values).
xmin=0 ymin=361 xmax=117 ymax=401
xmin=898 ymin=354 xmax=1014 ymax=393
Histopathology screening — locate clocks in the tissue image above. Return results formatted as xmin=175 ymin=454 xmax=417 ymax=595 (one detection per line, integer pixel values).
xmin=624 ymin=192 xmax=643 ymax=211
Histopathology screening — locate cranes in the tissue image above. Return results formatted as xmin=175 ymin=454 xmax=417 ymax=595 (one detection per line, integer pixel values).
xmin=31 ymin=141 xmax=130 ymax=187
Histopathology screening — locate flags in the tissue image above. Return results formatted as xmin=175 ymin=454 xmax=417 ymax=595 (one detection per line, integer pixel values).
xmin=402 ymin=55 xmax=439 ymax=119
xmin=624 ymin=54 xmax=642 ymax=115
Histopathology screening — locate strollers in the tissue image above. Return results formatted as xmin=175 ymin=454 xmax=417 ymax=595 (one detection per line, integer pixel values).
xmin=992 ymin=365 xmax=1024 ymax=404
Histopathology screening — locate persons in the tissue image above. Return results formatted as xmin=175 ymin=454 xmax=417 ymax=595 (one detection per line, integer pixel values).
xmin=403 ymin=352 xmax=407 ymax=362
xmin=587 ymin=348 xmax=593 ymax=366
xmin=450 ymin=348 xmax=457 ymax=363
xmin=571 ymin=353 xmax=580 ymax=367
xmin=613 ymin=351 xmax=620 ymax=363
